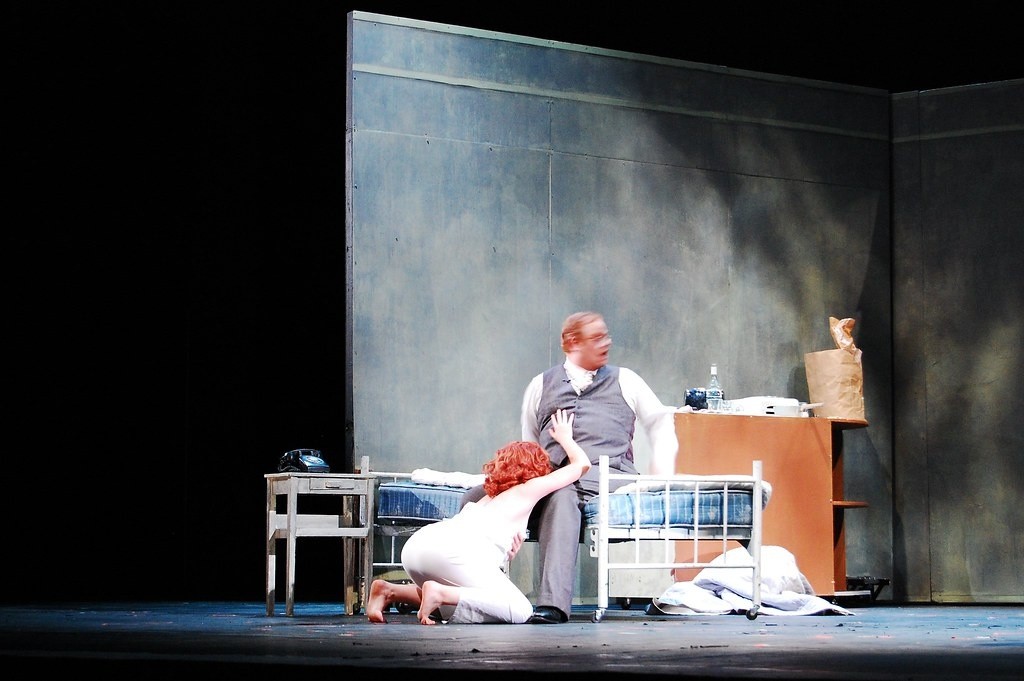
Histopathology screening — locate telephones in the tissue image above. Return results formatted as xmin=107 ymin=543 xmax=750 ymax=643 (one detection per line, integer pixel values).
xmin=282 ymin=447 xmax=331 ymax=472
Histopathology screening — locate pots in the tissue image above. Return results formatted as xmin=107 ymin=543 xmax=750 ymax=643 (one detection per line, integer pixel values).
xmin=764 ymin=403 xmax=824 ymax=417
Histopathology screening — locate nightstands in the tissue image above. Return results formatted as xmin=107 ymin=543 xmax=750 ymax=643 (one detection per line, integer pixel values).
xmin=264 ymin=472 xmax=377 ymax=616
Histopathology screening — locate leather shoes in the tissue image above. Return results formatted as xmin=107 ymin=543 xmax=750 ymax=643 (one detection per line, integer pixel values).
xmin=529 ymin=606 xmax=563 ymax=623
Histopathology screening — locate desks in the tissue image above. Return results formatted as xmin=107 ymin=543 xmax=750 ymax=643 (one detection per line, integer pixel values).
xmin=619 ymin=411 xmax=870 ymax=608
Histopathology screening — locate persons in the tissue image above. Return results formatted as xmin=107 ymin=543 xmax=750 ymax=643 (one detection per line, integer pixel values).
xmin=366 ymin=407 xmax=592 ymax=623
xmin=456 ymin=312 xmax=680 ymax=621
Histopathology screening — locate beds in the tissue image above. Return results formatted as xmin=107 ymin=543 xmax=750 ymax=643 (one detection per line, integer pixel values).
xmin=361 ymin=455 xmax=772 ymax=617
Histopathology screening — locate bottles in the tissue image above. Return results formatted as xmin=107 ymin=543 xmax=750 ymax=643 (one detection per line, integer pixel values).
xmin=706 ymin=363 xmax=723 ymax=415
